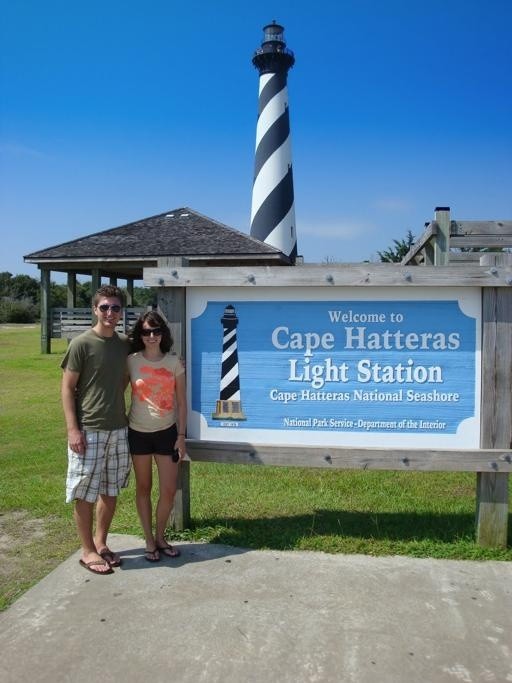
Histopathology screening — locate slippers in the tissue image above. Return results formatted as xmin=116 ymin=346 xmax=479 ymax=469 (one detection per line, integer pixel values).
xmin=143 ymin=544 xmax=181 ymax=562
xmin=78 ymin=550 xmax=123 ymax=575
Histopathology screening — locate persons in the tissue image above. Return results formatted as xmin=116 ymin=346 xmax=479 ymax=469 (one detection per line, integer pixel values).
xmin=124 ymin=310 xmax=189 ymax=564
xmin=60 ymin=281 xmax=133 ymax=577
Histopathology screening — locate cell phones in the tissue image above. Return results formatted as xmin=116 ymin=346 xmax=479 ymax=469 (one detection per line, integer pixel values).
xmin=172 ymin=449 xmax=180 ymax=463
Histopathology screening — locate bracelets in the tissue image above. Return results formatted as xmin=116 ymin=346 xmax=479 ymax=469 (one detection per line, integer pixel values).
xmin=177 ymin=432 xmax=186 ymax=436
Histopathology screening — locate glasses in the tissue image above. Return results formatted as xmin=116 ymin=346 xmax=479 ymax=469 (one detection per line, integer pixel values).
xmin=94 ymin=303 xmax=122 ymax=313
xmin=138 ymin=326 xmax=164 ymax=336
